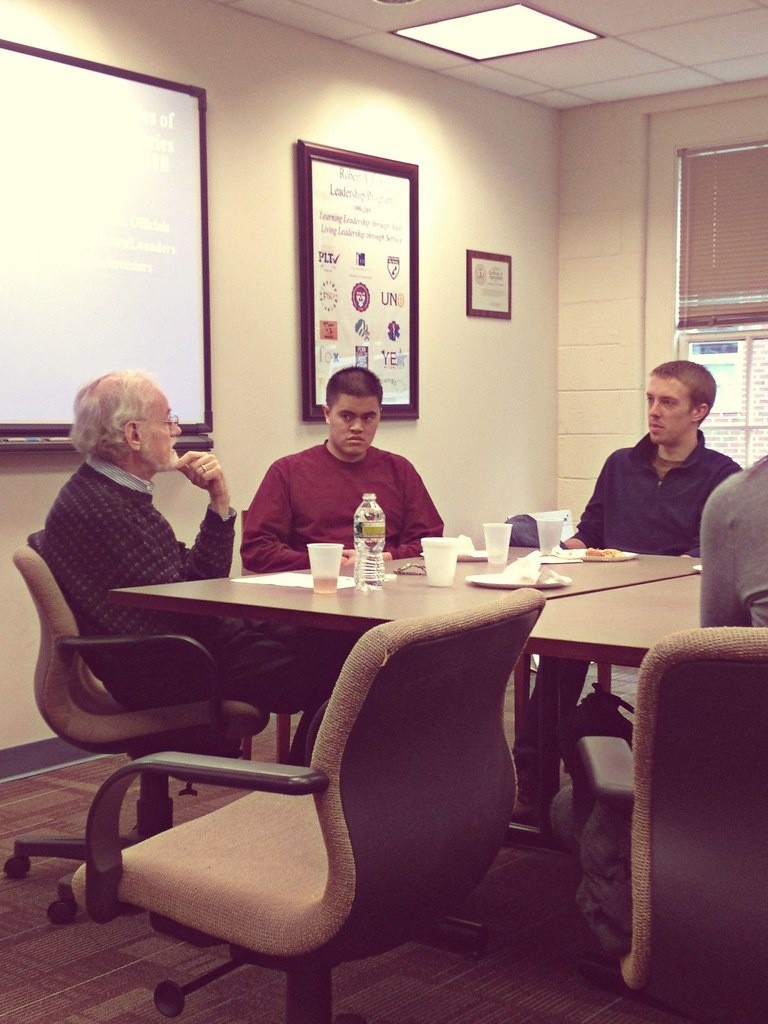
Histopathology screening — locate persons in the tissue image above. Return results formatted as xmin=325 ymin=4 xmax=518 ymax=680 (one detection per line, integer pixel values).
xmin=505 ymin=361 xmax=745 ymax=841
xmin=28 ymin=370 xmax=344 ymax=792
xmin=239 ymin=367 xmax=445 ymax=698
xmin=700 ymin=445 xmax=768 ymax=629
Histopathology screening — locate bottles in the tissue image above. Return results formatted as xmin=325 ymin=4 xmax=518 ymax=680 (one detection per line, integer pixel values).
xmin=352 ymin=492 xmax=385 ymax=593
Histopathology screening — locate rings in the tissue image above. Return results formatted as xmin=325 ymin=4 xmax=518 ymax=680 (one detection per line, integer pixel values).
xmin=200 ymin=465 xmax=207 ymax=473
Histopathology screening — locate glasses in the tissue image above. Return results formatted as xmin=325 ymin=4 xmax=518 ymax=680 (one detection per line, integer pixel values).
xmin=142 ymin=415 xmax=178 ymax=432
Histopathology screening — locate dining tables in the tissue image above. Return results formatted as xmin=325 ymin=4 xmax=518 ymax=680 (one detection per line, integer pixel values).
xmin=103 ymin=544 xmax=703 ymax=856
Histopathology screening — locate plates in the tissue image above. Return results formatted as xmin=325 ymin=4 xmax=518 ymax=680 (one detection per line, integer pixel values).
xmin=559 ymin=548 xmax=638 ymax=562
xmin=420 ymin=550 xmax=499 ymax=561
xmin=465 ymin=573 xmax=572 ymax=588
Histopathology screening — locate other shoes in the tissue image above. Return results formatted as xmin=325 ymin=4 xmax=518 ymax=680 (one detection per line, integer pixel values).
xmin=513 ymin=799 xmax=542 ymax=820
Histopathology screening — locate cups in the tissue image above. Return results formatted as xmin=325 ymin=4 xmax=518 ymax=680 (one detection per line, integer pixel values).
xmin=483 ymin=524 xmax=513 ymax=563
xmin=536 ymin=518 xmax=564 ymax=555
xmin=306 ymin=543 xmax=344 ymax=594
xmin=420 ymin=536 xmax=462 ymax=586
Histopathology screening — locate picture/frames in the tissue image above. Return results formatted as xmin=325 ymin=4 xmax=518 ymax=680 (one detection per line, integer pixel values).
xmin=295 ymin=139 xmax=419 ymax=422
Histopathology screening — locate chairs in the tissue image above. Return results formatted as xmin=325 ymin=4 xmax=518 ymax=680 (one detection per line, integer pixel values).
xmin=72 ymin=584 xmax=550 ymax=1022
xmin=5 ymin=534 xmax=270 ymax=922
xmin=571 ymin=624 xmax=768 ymax=1022
